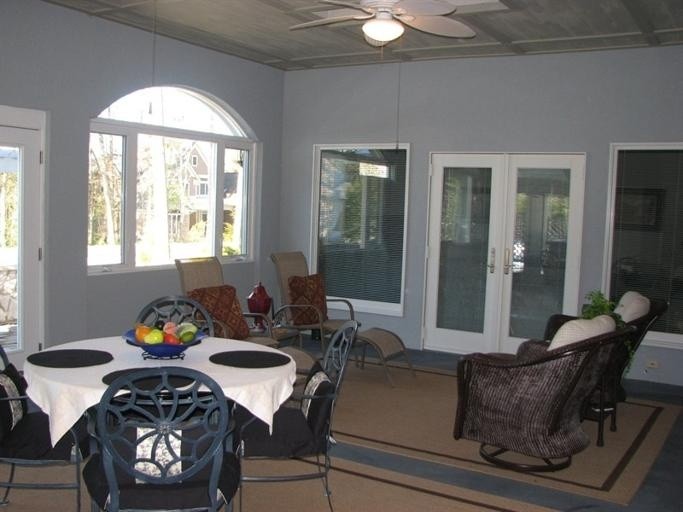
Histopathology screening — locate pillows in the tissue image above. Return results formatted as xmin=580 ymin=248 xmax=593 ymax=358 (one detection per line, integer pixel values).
xmin=287 ymin=274 xmax=328 ymax=326
xmin=186 ymin=285 xmax=250 ymax=339
xmin=613 ymin=289 xmax=651 ymax=323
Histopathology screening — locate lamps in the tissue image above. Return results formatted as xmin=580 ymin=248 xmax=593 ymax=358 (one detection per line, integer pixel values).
xmin=361 ymin=13 xmax=404 ymax=42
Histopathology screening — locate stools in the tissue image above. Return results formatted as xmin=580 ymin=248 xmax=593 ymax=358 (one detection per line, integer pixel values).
xmin=350 ymin=328 xmax=420 ymax=391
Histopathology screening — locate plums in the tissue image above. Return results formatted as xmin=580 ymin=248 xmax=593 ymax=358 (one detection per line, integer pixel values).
xmin=155 ymin=320 xmax=165 ymax=329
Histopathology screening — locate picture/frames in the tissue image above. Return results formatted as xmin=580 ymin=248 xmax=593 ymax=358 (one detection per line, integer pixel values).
xmin=615 ymin=186 xmax=663 ymax=229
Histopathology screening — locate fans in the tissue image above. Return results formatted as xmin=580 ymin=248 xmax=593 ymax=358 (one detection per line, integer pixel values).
xmin=289 ymin=0 xmax=477 ymax=47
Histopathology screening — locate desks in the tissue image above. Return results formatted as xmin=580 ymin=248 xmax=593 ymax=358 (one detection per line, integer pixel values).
xmin=22 ymin=333 xmax=297 ymax=450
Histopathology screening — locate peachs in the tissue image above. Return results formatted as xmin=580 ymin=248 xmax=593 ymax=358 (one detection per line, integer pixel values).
xmin=163 ymin=321 xmax=177 ymax=334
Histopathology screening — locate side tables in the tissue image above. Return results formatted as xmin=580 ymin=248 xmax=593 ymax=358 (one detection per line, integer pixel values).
xmin=581 ymin=369 xmax=616 ymax=447
xmin=249 ymin=325 xmax=303 ymax=351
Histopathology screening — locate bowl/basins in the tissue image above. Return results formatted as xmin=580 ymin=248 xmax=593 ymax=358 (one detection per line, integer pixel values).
xmin=122 ymin=326 xmax=204 ymax=354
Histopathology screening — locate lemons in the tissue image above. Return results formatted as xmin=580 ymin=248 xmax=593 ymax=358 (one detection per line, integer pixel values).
xmin=181 ymin=331 xmax=194 ymax=342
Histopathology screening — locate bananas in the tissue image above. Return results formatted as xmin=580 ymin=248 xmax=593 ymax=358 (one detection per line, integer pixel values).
xmin=176 ymin=323 xmax=197 ymax=338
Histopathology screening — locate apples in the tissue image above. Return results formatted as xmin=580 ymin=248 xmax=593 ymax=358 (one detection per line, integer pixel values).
xmin=165 ymin=334 xmax=181 ymax=345
xmin=142 ymin=330 xmax=163 ymax=344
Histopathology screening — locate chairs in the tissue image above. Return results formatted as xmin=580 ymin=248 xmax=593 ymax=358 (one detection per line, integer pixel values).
xmin=543 ymin=300 xmax=670 ymax=403
xmin=269 ymin=250 xmax=355 ymax=357
xmin=0 ymin=342 xmax=89 ymax=512
xmin=82 ymin=365 xmax=241 ymax=512
xmin=453 ymin=324 xmax=638 ymax=473
xmin=136 ymin=296 xmax=215 ymax=338
xmin=174 ymin=255 xmax=281 ymax=349
xmin=233 ymin=320 xmax=358 ymax=512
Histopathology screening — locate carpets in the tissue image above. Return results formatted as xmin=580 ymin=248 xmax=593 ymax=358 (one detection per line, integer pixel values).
xmin=281 ymin=342 xmax=683 ymax=507
xmin=0 ymin=453 xmax=563 ymax=512
